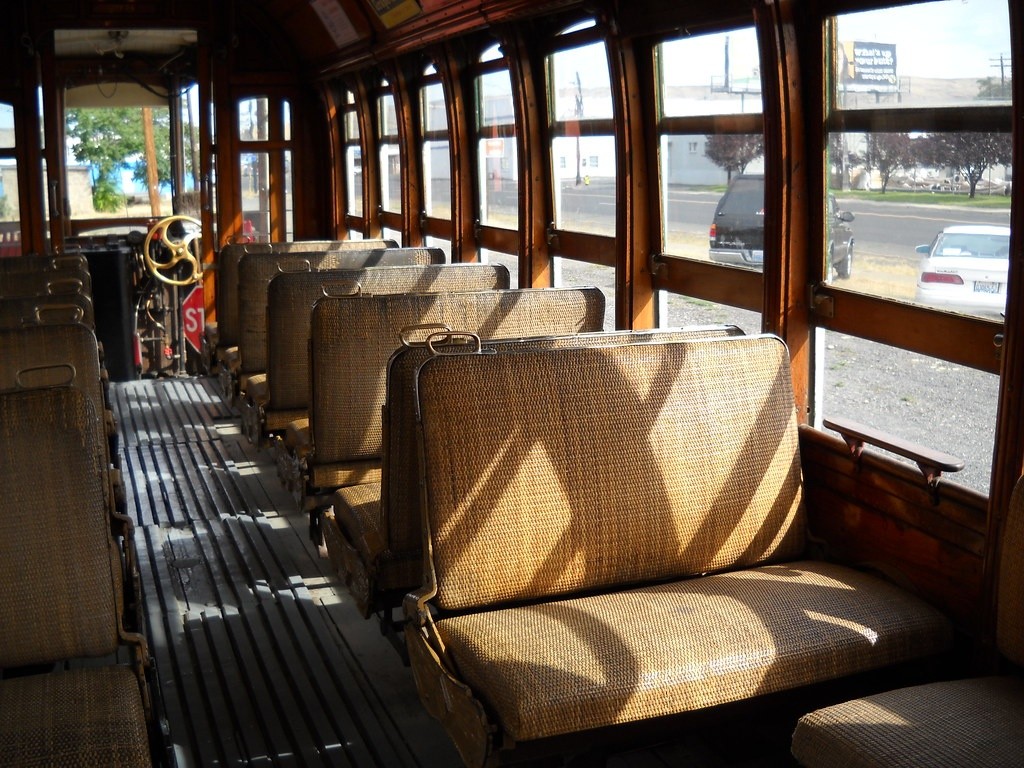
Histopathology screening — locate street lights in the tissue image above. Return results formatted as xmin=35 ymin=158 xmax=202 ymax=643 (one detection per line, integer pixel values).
xmin=574 ymin=71 xmax=584 ymax=186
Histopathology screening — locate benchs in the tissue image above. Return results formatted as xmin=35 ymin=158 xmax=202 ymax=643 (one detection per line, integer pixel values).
xmin=0 ymin=239 xmax=187 ymax=768
xmin=202 ymin=249 xmax=1024 ymax=768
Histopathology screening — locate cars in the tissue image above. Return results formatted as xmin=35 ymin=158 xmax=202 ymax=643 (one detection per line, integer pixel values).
xmin=914 ymin=225 xmax=1010 ymax=322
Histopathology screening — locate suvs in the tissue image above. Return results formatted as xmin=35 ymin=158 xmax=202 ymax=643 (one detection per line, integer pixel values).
xmin=708 ymin=174 xmax=855 ymax=286
